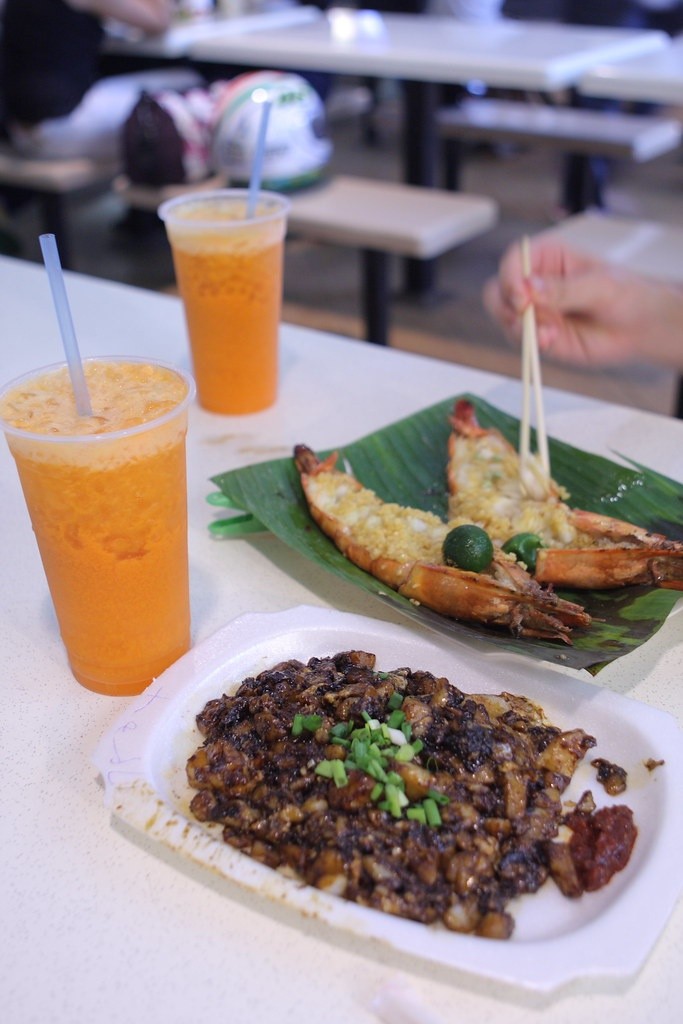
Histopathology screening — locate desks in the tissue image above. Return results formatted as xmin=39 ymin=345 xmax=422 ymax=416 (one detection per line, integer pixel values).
xmin=574 ymin=35 xmax=683 ymax=107
xmin=106 ymin=6 xmax=323 ymax=56
xmin=188 ymin=12 xmax=670 ymax=312
xmin=0 ymin=254 xmax=683 ymax=1024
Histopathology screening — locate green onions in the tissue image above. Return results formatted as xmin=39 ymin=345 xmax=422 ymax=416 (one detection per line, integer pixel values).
xmin=291 ymin=673 xmax=449 ymax=827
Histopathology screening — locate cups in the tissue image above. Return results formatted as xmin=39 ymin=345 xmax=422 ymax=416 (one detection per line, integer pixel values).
xmin=158 ymin=187 xmax=292 ymax=415
xmin=0 ymin=355 xmax=197 ymax=697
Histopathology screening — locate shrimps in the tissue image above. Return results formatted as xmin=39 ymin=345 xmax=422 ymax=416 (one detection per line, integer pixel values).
xmin=294 ymin=399 xmax=682 ymax=647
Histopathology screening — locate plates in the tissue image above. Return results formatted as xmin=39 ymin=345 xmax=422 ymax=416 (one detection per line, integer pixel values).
xmin=92 ymin=604 xmax=683 ymax=992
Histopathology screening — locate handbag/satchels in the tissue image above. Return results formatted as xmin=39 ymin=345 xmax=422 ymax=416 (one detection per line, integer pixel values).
xmin=119 ymin=85 xmax=216 ymax=187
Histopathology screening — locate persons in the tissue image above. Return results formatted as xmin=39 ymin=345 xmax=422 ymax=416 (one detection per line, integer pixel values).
xmin=483 ymin=207 xmax=683 ymax=420
xmin=0 ymin=1 xmax=204 ymax=257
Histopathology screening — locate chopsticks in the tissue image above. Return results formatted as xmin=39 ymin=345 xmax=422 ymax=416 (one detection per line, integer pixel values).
xmin=519 ymin=232 xmax=552 ymax=486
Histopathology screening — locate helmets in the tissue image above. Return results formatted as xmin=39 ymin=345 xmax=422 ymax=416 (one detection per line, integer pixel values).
xmin=211 ymin=72 xmax=334 ymax=190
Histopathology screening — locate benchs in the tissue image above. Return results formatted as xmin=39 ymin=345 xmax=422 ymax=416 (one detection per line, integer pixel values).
xmin=377 ymin=96 xmax=681 ymax=219
xmin=113 ymin=170 xmax=499 ymax=347
xmin=0 ymin=151 xmax=114 ymax=272
